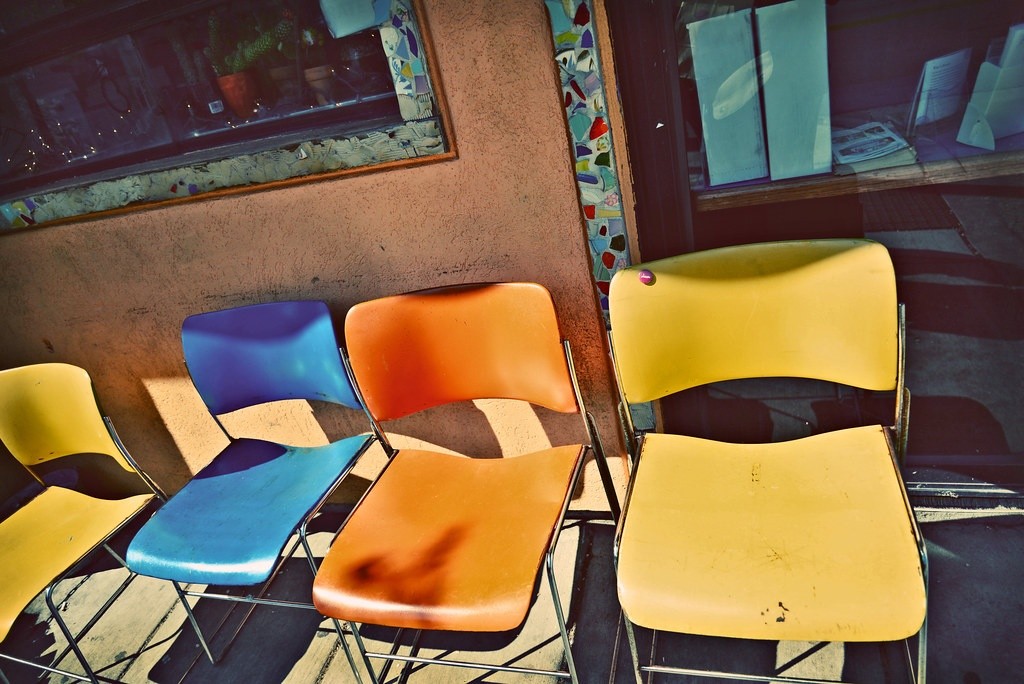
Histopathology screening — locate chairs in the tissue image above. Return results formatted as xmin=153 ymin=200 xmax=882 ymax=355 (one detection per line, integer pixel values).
xmin=313 ymin=280 xmax=624 ymax=683
xmin=607 ymin=237 xmax=930 ymax=684
xmin=126 ymin=297 xmax=408 ymax=684
xmin=0 ymin=361 xmax=249 ymax=684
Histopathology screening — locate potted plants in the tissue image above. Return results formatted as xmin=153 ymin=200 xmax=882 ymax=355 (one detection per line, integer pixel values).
xmin=165 ymin=0 xmax=339 ymax=119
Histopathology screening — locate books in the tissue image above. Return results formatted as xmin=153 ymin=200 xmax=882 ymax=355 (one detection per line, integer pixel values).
xmin=1000 ymin=23 xmax=1024 ymax=68
xmin=831 ymin=120 xmax=915 ymax=178
